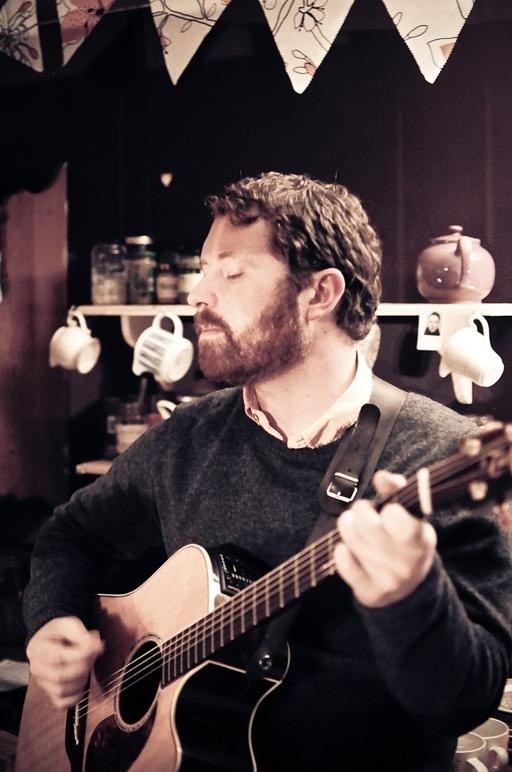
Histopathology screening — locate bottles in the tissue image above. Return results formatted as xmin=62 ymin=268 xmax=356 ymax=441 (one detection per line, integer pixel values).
xmin=91 ymin=233 xmax=200 ymax=300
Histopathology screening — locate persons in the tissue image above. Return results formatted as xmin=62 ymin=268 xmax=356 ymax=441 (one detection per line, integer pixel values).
xmin=424 ymin=311 xmax=440 ymax=335
xmin=18 ymin=171 xmax=512 ymax=771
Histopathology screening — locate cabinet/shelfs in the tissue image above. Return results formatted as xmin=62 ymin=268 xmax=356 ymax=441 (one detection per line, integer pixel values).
xmin=70 ymin=299 xmax=512 ymax=478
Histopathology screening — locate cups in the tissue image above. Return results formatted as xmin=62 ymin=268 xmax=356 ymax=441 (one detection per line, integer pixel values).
xmin=133 ymin=308 xmax=196 ymax=382
xmin=437 ymin=313 xmax=504 ymax=388
xmin=453 ymin=715 xmax=509 ymax=772
xmin=48 ymin=311 xmax=103 ymax=377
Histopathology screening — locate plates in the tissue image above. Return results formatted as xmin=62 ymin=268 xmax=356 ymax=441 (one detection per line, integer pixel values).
xmin=498 ymin=678 xmax=512 ymax=712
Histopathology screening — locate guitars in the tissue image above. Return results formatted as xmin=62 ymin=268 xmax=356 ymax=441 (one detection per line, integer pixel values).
xmin=15 ymin=418 xmax=510 ymax=770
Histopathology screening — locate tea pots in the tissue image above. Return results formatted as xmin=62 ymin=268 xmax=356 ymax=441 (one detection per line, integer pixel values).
xmin=412 ymin=222 xmax=495 ymax=302
